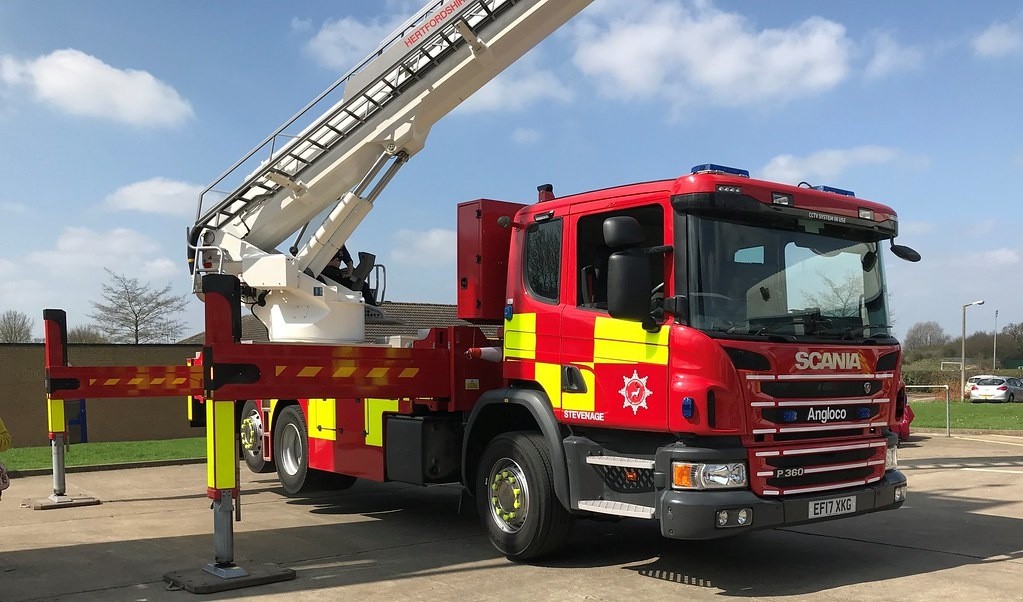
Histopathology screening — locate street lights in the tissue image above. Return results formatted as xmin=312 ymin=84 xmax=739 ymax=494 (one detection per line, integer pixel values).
xmin=961 ymin=301 xmax=984 ymax=402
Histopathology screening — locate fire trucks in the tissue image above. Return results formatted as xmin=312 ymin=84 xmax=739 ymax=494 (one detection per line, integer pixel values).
xmin=21 ymin=0 xmax=923 ymax=594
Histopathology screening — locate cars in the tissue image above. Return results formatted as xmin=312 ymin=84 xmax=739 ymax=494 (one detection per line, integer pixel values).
xmin=963 ymin=375 xmax=998 ymax=394
xmin=970 ymin=377 xmax=1023 ymax=403
